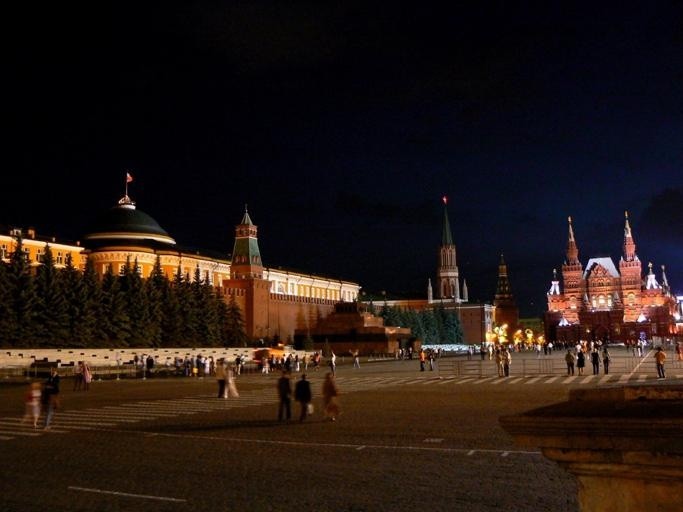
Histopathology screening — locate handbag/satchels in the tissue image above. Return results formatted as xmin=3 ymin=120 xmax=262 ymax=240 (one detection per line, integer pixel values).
xmin=305 ymin=402 xmax=314 ymax=416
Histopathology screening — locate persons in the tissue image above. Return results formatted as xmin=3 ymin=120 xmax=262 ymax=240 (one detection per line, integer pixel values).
xmin=19 ymin=336 xmax=683 ymax=433
